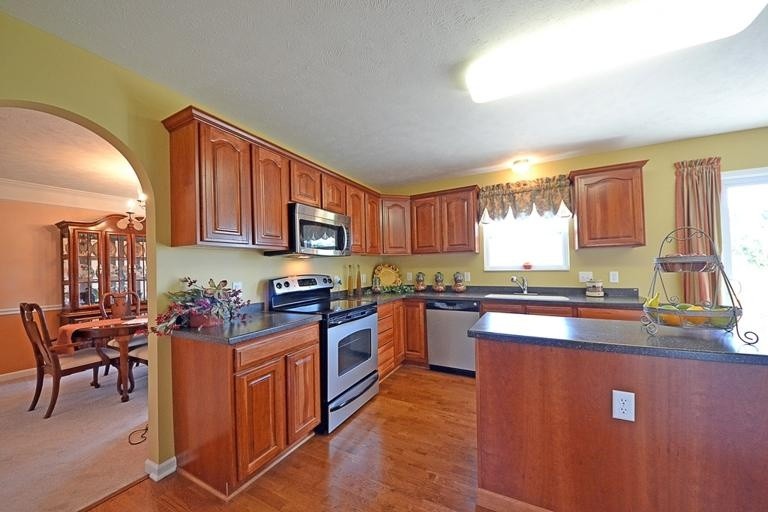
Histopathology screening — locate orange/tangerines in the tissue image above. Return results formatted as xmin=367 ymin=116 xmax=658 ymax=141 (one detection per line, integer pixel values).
xmin=661 ymin=304 xmax=736 ymax=326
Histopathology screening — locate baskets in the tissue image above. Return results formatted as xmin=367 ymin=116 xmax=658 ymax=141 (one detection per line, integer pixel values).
xmin=654 ymin=256 xmax=721 ymax=272
xmin=643 ymin=303 xmax=741 ymax=329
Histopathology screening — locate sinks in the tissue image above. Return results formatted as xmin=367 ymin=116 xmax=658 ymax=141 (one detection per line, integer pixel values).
xmin=484 ymin=294 xmax=571 ymax=302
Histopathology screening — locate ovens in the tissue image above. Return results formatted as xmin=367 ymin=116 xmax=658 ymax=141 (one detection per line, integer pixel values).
xmin=320 ymin=313 xmax=380 ymax=435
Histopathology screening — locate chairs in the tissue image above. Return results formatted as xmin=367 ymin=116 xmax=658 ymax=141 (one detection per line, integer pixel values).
xmin=19 ymin=303 xmax=104 ymax=419
xmin=99 ymin=290 xmax=148 ymax=393
xmin=128 ymin=345 xmax=149 ymax=393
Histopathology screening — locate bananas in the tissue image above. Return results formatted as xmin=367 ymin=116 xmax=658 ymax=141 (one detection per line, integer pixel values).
xmin=643 ymin=291 xmax=660 ymax=319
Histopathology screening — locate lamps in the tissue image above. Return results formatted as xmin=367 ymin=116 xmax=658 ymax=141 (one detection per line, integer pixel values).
xmin=496 ymin=151 xmax=542 ymax=177
xmin=116 ymin=193 xmax=148 ymax=232
xmin=460 ymin=0 xmax=768 ymax=106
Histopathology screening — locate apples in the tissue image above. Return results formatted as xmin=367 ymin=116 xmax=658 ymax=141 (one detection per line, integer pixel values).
xmin=662 ymin=253 xmax=708 ymax=272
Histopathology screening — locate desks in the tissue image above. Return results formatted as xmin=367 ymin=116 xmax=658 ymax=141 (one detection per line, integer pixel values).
xmin=71 ymin=313 xmax=148 ymax=402
xmin=466 ymin=310 xmax=768 ymax=512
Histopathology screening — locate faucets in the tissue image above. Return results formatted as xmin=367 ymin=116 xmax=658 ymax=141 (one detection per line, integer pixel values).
xmin=511 ymin=275 xmax=529 ymax=294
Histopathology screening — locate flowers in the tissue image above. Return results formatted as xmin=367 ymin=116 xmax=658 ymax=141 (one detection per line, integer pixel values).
xmin=133 ymin=275 xmax=251 ymax=338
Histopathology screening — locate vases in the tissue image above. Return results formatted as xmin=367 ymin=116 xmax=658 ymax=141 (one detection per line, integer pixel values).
xmin=190 ymin=312 xmax=224 ymax=327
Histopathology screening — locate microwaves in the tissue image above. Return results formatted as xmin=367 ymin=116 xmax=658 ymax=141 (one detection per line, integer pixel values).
xmin=289 ymin=203 xmax=351 ymax=257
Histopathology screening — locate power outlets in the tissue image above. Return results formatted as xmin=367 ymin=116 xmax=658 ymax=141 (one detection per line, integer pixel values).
xmin=611 ymin=389 xmax=636 ymax=422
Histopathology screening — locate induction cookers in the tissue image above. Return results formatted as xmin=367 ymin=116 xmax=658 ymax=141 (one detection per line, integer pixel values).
xmin=275 ymin=297 xmax=378 ymax=326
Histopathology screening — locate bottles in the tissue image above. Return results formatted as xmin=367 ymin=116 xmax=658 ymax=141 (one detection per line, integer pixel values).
xmin=373 ymin=274 xmax=380 ymax=286
xmin=348 ymin=264 xmax=361 ymax=296
xmin=415 ymin=272 xmax=465 ymax=292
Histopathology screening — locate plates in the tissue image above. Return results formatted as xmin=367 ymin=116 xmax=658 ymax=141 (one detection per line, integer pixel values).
xmin=374 ymin=264 xmax=401 ymax=289
xmin=79 ymin=240 xmax=145 ymax=302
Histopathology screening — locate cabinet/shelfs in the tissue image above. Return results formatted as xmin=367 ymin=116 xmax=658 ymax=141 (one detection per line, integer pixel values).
xmin=290 ymin=159 xmax=347 ymax=214
xmin=54 ymin=212 xmax=147 ymax=326
xmin=171 ymin=321 xmax=324 ymax=495
xmin=382 ymin=197 xmax=411 ymax=254
xmin=345 ymin=181 xmax=383 ymax=255
xmin=479 ymin=302 xmax=643 ymax=321
xmin=376 ymin=299 xmax=429 ymax=385
xmin=568 ymin=158 xmax=650 ymax=247
xmin=410 ymin=196 xmax=442 ymax=254
xmin=440 ymin=191 xmax=475 ymax=252
xmin=249 ymin=138 xmax=291 ymax=251
xmin=163 ymin=106 xmax=250 ymax=249
